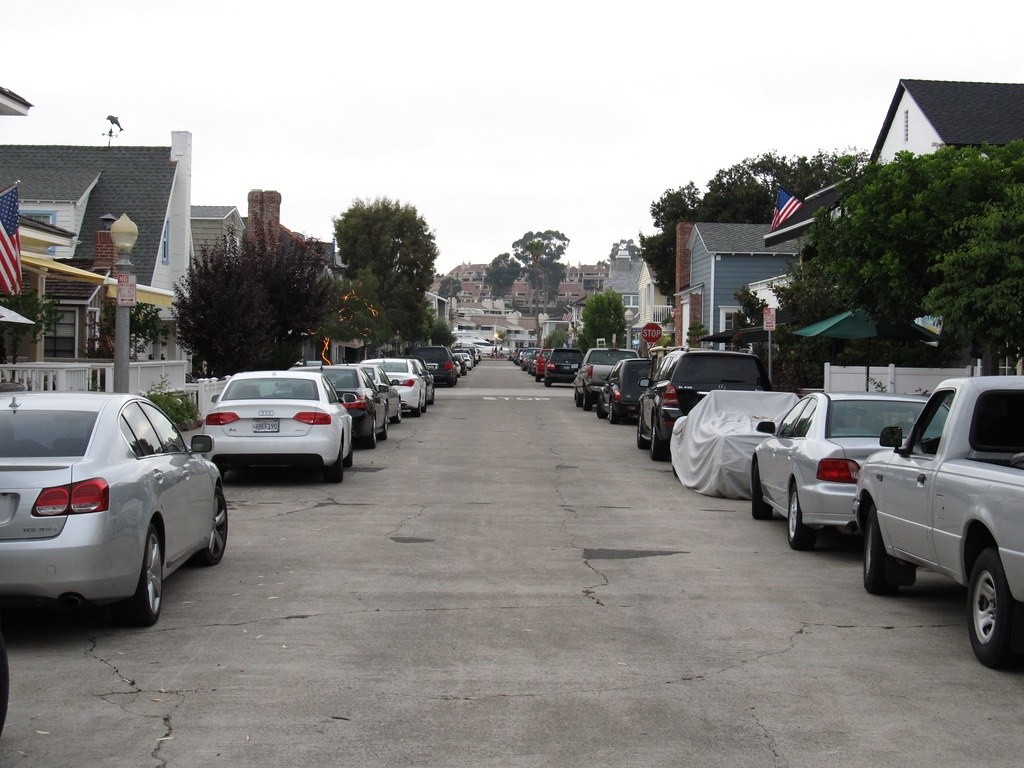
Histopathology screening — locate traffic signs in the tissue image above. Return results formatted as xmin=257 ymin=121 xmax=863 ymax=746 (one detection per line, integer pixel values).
xmin=641 ymin=323 xmax=662 ymax=347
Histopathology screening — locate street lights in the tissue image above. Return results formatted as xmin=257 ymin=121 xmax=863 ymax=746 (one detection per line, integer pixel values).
xmin=109 ymin=212 xmax=138 ymax=394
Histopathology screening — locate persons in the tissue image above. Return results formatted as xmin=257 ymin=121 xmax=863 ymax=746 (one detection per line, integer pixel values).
xmin=490 ymin=347 xmax=505 ymax=360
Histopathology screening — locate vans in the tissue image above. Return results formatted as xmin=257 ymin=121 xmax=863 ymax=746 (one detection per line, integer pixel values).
xmin=413 ymin=346 xmax=457 ymax=387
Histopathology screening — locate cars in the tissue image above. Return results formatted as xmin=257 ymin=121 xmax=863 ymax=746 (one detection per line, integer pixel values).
xmin=198 ymin=369 xmax=354 ymax=484
xmin=750 ymin=389 xmax=953 ymax=551
xmin=448 ymin=340 xmax=585 ymax=387
xmin=597 ymin=356 xmax=652 ymax=423
xmin=403 ymin=356 xmax=435 ymax=406
xmin=288 ymin=366 xmax=390 ymax=449
xmin=360 ymin=358 xmax=427 ymax=416
xmin=0 ymin=389 xmax=228 ymax=626
xmin=335 ymin=363 xmax=403 ymax=424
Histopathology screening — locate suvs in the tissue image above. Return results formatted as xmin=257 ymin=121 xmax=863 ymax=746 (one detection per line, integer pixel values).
xmin=637 ymin=346 xmax=775 ymax=461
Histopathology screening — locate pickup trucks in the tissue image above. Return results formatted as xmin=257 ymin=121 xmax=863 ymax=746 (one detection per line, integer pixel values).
xmin=572 ymin=346 xmax=640 ymax=411
xmin=856 ymin=374 xmax=1024 ymax=671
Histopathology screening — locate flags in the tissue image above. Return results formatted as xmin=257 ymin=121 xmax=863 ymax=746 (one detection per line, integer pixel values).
xmin=770 ymin=185 xmax=805 ymax=233
xmin=0 ymin=179 xmax=24 ymax=296
